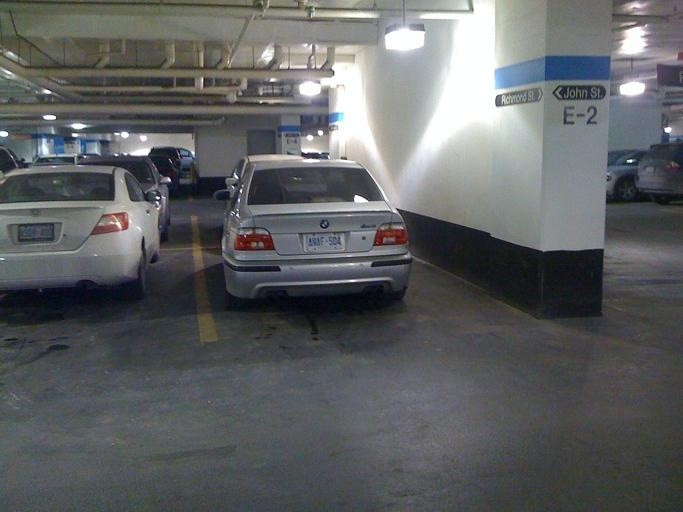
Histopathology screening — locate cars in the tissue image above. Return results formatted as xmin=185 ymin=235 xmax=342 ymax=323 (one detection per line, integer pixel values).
xmin=0 ymin=143 xmax=197 ymax=299
xmin=636 ymin=142 xmax=683 ymax=204
xmin=213 ymin=151 xmax=413 ymax=311
xmin=606 ymin=147 xmax=647 ymax=203
